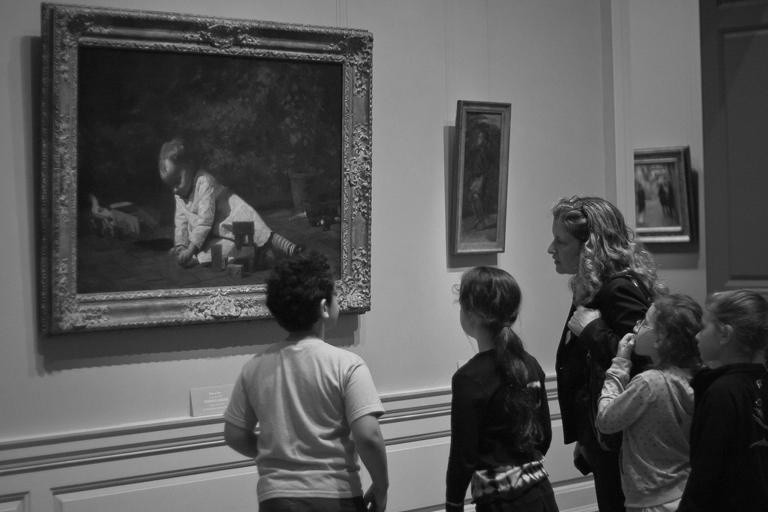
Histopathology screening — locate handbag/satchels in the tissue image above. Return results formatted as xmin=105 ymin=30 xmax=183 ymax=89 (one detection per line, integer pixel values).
xmin=588 ymin=367 xmax=643 ymax=452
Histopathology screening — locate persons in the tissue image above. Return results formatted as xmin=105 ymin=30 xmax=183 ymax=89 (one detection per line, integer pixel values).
xmin=156 ymin=133 xmax=303 ymax=269
xmin=443 ymin=265 xmax=560 ymax=511
xmin=224 ymin=250 xmax=389 ymax=510
xmin=635 ymin=177 xmax=677 ymax=223
xmin=689 ymin=289 xmax=768 ymax=511
xmin=547 ymin=195 xmax=669 ymax=512
xmin=593 ymin=294 xmax=701 ymax=512
xmin=464 ymin=125 xmax=492 ymax=231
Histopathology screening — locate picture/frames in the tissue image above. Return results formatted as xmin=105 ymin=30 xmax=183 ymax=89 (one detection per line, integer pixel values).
xmin=452 ymin=100 xmax=509 ymax=254
xmin=633 ymin=146 xmax=693 ymax=246
xmin=38 ymin=4 xmax=372 ymax=332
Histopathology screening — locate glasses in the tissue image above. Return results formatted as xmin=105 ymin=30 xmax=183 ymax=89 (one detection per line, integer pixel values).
xmin=569 ymin=194 xmax=588 ymax=218
xmin=636 ymin=319 xmax=653 ymax=333
